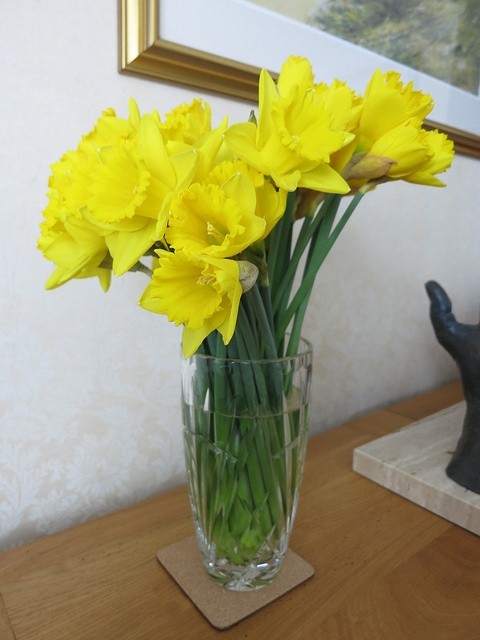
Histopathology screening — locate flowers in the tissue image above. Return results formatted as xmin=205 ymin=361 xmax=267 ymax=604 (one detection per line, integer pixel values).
xmin=39 ymin=55 xmax=458 ymax=361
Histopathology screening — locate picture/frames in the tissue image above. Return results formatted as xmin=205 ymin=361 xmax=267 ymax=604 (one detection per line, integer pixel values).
xmin=116 ymin=1 xmax=479 ymax=161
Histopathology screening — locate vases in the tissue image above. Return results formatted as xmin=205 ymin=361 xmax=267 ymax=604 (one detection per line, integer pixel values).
xmin=177 ymin=333 xmax=313 ymax=591
